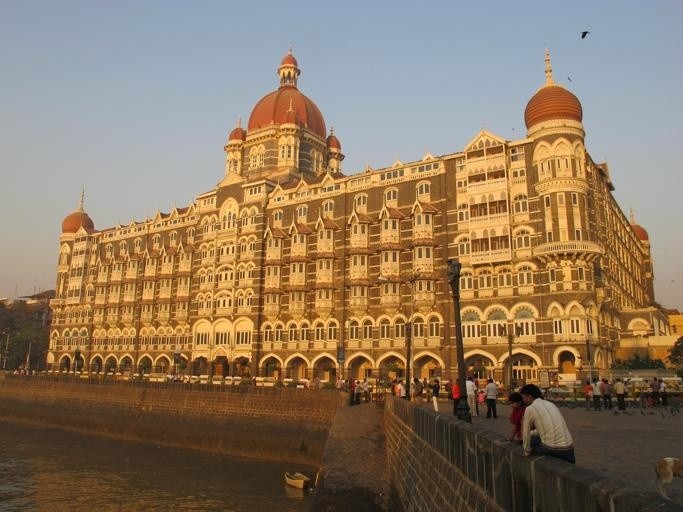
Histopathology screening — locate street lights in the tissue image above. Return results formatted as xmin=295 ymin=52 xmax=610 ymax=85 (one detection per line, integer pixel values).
xmin=27 ymin=340 xmax=37 ymax=364
xmin=447 ymin=258 xmax=471 ymax=423
xmin=3 ymin=332 xmax=10 ymax=368
xmin=405 ymin=323 xmax=411 ymax=400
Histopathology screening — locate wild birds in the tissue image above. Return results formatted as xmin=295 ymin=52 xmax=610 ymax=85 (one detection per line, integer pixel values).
xmin=580 ymin=31 xmax=589 ymax=40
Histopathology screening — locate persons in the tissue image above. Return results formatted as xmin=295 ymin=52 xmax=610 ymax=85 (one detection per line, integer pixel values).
xmin=170 ymin=372 xmax=201 ymax=383
xmin=335 ymin=376 xmax=498 ymax=419
xmin=231 ymin=375 xmax=235 ymax=384
xmin=505 ymin=392 xmax=535 ymax=443
xmin=519 ymin=383 xmax=575 ymax=464
xmin=252 ymin=376 xmax=257 ymax=385
xmin=306 ymin=378 xmax=310 ymax=388
xmin=584 ymin=376 xmax=669 ymax=410
xmin=13 ymin=368 xmax=36 ymax=375
xmin=314 ymin=376 xmax=320 ymax=389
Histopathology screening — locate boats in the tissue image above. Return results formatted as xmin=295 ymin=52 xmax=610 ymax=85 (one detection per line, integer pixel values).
xmin=285 ymin=472 xmax=310 ymax=488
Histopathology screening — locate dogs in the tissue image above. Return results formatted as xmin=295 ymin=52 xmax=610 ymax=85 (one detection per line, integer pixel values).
xmin=653 ymin=456 xmax=683 ymax=501
